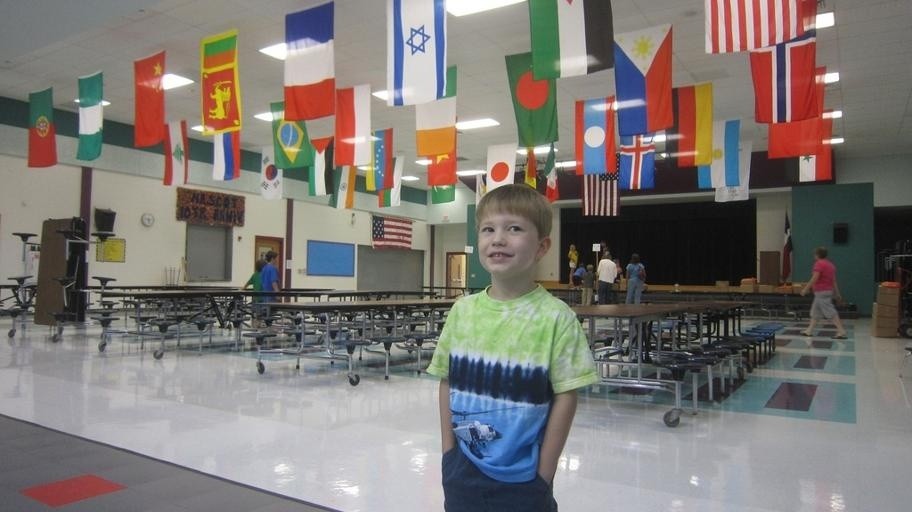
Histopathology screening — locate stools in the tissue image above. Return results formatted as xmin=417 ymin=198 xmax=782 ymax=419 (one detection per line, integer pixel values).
xmin=0 ymin=268 xmax=486 ymax=388
xmin=540 ymin=277 xmax=842 ymax=428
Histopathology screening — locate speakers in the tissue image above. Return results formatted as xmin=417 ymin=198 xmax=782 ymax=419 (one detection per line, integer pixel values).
xmin=94 ymin=208 xmax=116 ymax=231
xmin=834 ymin=222 xmax=848 ymax=244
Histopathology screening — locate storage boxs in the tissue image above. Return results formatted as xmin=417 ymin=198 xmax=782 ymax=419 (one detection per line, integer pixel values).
xmin=871 ymin=282 xmax=900 ymax=339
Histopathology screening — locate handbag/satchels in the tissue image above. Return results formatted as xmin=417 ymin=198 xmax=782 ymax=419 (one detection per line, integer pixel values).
xmin=638 ymin=265 xmax=645 ymax=280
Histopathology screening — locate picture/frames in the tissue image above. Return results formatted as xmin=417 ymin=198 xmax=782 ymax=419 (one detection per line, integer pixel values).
xmin=305 ymin=238 xmax=357 ymax=278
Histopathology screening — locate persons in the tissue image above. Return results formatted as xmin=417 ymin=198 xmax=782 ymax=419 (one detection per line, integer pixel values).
xmin=800 ymin=246 xmax=849 ymax=338
xmin=242 ymin=261 xmax=266 ymax=328
xmin=426 ymin=183 xmax=600 ymax=511
xmin=261 ymin=252 xmax=283 ymax=327
xmin=568 ymin=241 xmax=647 ymax=306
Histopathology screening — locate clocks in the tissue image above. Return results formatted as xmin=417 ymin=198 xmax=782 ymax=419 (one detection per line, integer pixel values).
xmin=141 ymin=213 xmax=156 ymax=228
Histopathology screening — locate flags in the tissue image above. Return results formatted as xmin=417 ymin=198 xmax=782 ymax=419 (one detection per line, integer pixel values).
xmin=212 ymin=132 xmax=240 ymax=180
xmin=164 ymin=118 xmax=189 ymax=186
xmin=75 ymin=71 xmax=103 ymax=164
xmin=133 ymin=49 xmax=165 ymax=148
xmin=27 ymin=87 xmax=56 ymax=168
xmin=199 ymin=29 xmax=243 ymax=136
xmin=370 ymin=215 xmax=413 ymax=250
xmin=782 ymin=211 xmax=794 ymax=279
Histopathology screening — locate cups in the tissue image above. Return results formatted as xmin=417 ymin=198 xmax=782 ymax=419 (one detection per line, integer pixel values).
xmin=674 ymin=284 xmax=680 ymax=291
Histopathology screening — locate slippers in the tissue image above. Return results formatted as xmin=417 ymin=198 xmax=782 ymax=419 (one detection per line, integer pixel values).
xmin=831 ymin=335 xmax=848 ymax=339
xmin=799 ymin=329 xmax=813 ymax=336
